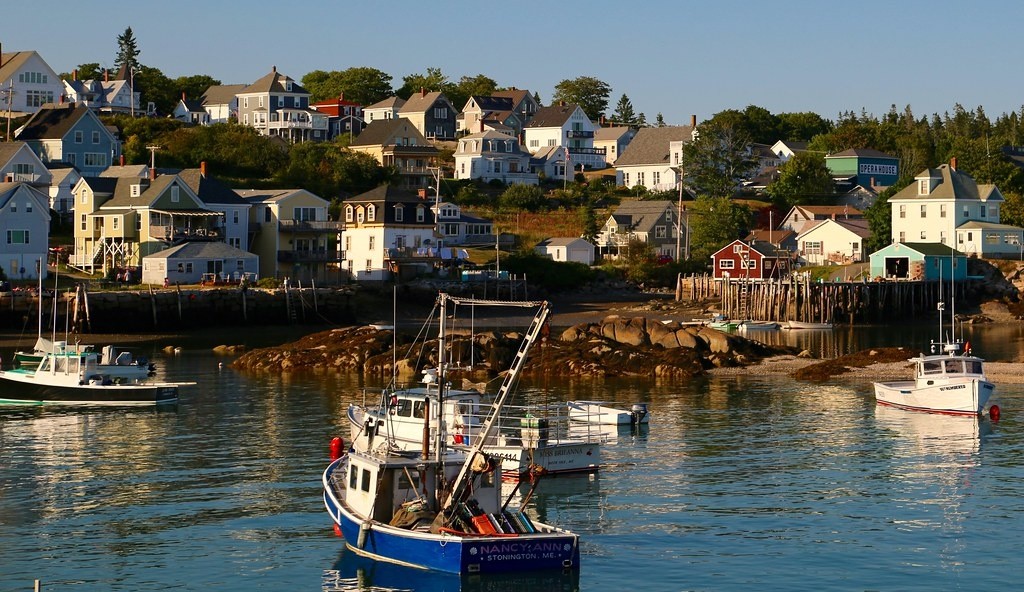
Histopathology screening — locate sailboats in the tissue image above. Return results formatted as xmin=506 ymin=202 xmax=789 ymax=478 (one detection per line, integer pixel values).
xmin=872 ymin=250 xmax=996 ymax=415
xmin=0 ymin=247 xmax=197 ymax=408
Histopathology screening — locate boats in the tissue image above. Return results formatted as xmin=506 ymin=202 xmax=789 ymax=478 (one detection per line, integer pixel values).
xmin=322 ymin=286 xmax=616 ymax=577
xmin=662 ymin=317 xmax=834 ymax=330
xmin=566 ymin=398 xmax=652 ymax=425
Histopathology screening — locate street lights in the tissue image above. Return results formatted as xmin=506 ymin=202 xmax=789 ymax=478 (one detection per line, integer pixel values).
xmin=428 ymin=184 xmax=439 ymax=223
xmin=131 ymin=67 xmax=142 ymax=117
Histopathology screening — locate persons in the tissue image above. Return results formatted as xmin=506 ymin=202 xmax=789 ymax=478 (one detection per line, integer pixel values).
xmin=117 ymin=269 xmax=131 ymax=289
